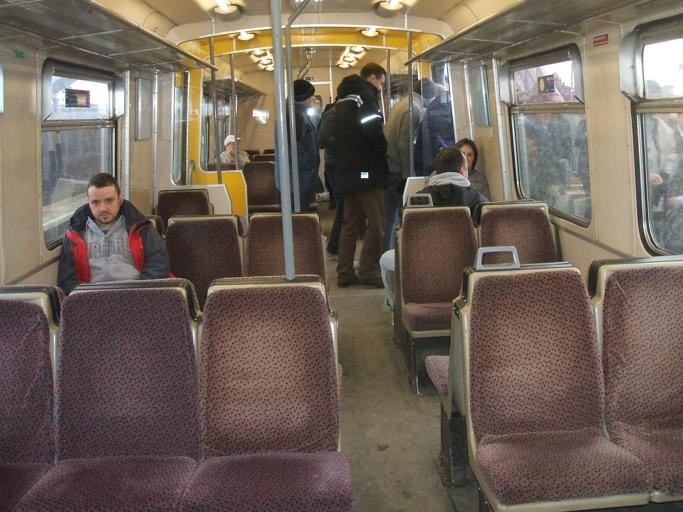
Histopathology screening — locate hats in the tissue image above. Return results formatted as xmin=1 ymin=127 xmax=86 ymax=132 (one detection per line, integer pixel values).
xmin=224 ymin=135 xmax=241 ymax=146
xmin=413 ymin=78 xmax=438 ymax=98
xmin=294 ymin=79 xmax=314 ymax=102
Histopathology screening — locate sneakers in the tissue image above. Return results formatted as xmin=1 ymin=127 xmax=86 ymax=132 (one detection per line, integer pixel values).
xmin=338 ymin=274 xmax=385 ymax=288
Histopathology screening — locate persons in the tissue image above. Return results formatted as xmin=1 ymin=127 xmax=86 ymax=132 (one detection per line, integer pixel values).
xmin=379 ymin=144 xmax=492 ymax=313
xmin=271 ymin=61 xmax=440 ymax=288
xmin=513 ymin=63 xmax=683 ymax=253
xmin=212 ymin=134 xmax=249 ymax=166
xmin=54 ymin=171 xmax=179 ymax=301
